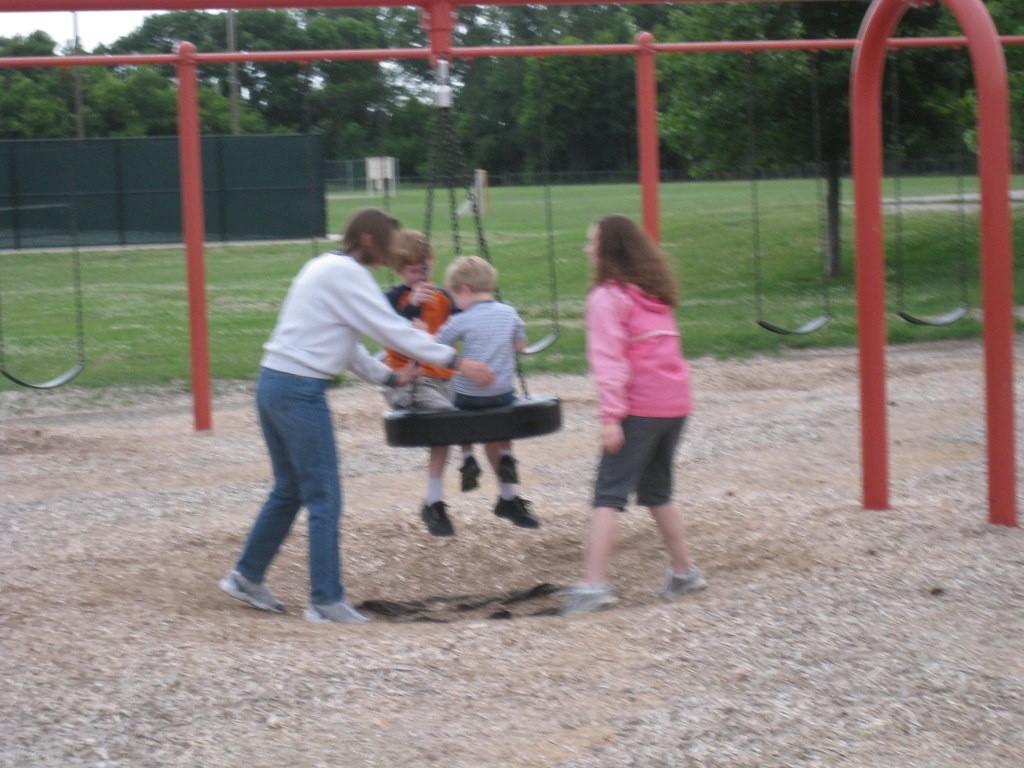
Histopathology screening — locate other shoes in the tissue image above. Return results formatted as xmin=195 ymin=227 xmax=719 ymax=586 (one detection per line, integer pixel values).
xmin=498 ymin=453 xmax=518 ymax=484
xmin=460 ymin=456 xmax=481 ymax=492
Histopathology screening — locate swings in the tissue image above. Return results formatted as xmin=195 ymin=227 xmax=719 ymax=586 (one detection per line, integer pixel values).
xmin=380 ymin=60 xmax=566 ymax=452
xmin=464 ymin=57 xmax=562 ymax=359
xmin=889 ymin=45 xmax=971 ymax=329
xmin=742 ymin=51 xmax=835 ymax=337
xmin=0 ymin=65 xmax=88 ymax=392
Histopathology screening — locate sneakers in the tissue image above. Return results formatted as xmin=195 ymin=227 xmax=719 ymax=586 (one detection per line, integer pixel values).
xmin=302 ymin=602 xmax=369 ymax=624
xmin=495 ymin=496 xmax=540 ymax=529
xmin=654 ymin=569 xmax=708 ymax=603
xmin=559 ymin=587 xmax=617 ymax=617
xmin=421 ymin=501 xmax=454 ymax=536
xmin=218 ymin=570 xmax=286 ymax=612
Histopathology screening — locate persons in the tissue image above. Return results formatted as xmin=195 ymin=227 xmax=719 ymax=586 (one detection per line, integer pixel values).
xmin=554 ymin=216 xmax=708 ymax=611
xmin=219 ymin=209 xmax=496 ymax=624
xmin=383 ymin=229 xmax=540 ymax=536
